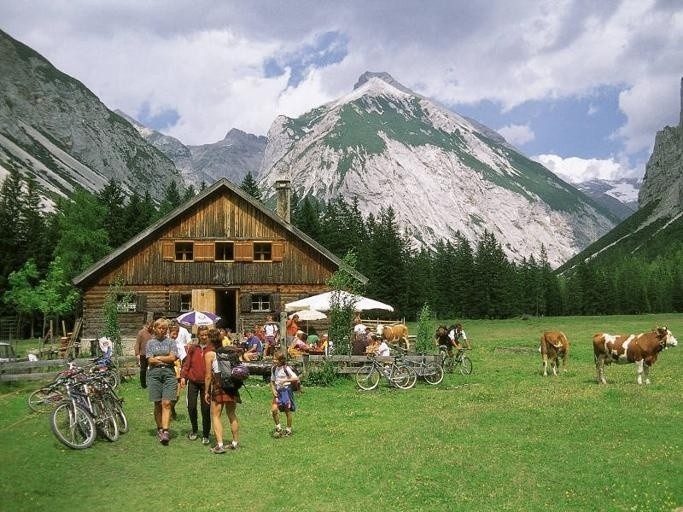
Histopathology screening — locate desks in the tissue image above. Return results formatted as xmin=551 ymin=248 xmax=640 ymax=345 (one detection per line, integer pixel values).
xmin=305 ymin=346 xmax=326 ymax=356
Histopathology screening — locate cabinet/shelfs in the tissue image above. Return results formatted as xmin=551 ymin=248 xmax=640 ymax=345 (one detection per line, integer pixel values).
xmin=81 ymin=338 xmax=98 ymax=356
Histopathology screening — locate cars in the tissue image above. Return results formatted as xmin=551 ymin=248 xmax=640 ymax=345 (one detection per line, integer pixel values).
xmin=0 ymin=342 xmax=20 ymax=362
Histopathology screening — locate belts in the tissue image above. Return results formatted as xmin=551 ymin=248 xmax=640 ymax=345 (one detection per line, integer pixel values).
xmin=154 ymin=363 xmax=172 ymax=369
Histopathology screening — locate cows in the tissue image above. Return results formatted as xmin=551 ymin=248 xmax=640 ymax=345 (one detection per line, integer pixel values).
xmin=375 ymin=323 xmax=409 ymax=350
xmin=592 ymin=324 xmax=678 ymax=386
xmin=538 ymin=331 xmax=569 ymax=378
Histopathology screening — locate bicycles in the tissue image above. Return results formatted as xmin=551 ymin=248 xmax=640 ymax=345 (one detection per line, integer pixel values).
xmin=441 ymin=346 xmax=473 ymax=375
xmin=354 ymin=347 xmax=444 ymax=389
xmin=27 ymin=353 xmax=132 ymax=449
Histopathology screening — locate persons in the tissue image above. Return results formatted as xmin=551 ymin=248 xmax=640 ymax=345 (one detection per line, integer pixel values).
xmin=203 ymin=329 xmax=242 ymax=454
xmin=268 ymin=350 xmax=299 ymax=437
xmin=178 ymin=325 xmax=211 ymax=445
xmin=144 ymin=317 xmax=178 ymax=443
xmin=133 ymin=312 xmax=409 ymax=419
xmin=439 ymin=323 xmax=472 ymax=373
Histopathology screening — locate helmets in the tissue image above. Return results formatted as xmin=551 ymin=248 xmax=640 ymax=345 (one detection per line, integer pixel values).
xmin=232 ymin=365 xmax=250 ymax=380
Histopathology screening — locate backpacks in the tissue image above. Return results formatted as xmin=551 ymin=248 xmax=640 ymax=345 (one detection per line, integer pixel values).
xmin=438 ymin=323 xmax=457 ymax=335
xmin=284 ymin=365 xmax=301 ymax=391
xmin=216 ymin=346 xmax=242 ymax=392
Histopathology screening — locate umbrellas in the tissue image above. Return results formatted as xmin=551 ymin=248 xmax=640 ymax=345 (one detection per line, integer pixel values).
xmin=285 ymin=287 xmax=393 ymax=334
xmin=288 ymin=307 xmax=326 ymax=336
xmin=176 ymin=307 xmax=221 ymax=338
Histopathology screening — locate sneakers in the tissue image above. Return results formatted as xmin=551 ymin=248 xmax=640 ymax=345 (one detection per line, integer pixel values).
xmin=212 ymin=444 xmax=227 ymax=454
xmin=202 ymin=436 xmax=210 ymax=445
xmin=271 ymin=429 xmax=284 ymax=437
xmin=283 ymin=426 xmax=293 ymax=437
xmin=188 ymin=432 xmax=199 ymax=441
xmin=226 ymin=440 xmax=241 ymax=451
xmin=156 ymin=428 xmax=170 ymax=445
